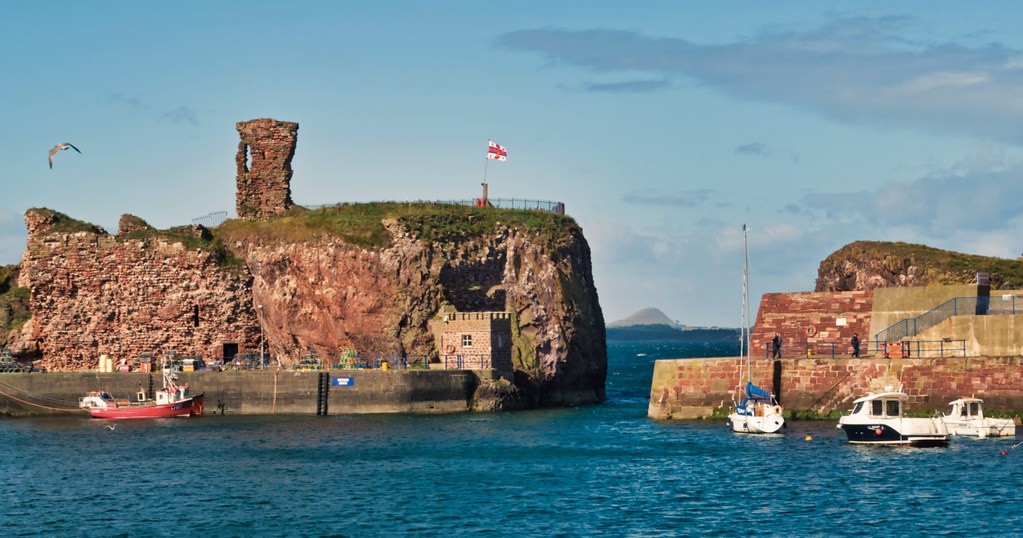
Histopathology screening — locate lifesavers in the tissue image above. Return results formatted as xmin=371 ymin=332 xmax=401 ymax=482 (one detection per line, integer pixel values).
xmin=89 ymin=391 xmax=99 ymax=397
xmin=805 ymin=324 xmax=816 ymax=337
xmin=772 ymin=405 xmax=782 ymax=415
xmin=445 ymin=342 xmax=455 ymax=353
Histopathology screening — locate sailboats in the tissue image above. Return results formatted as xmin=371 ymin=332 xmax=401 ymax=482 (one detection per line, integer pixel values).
xmin=727 ymin=224 xmax=788 ymax=434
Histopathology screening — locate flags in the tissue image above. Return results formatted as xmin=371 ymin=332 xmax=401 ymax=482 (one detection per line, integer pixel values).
xmin=486 ymin=140 xmax=507 ymax=161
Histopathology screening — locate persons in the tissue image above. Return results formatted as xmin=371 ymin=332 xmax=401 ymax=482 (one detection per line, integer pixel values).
xmin=771 ymin=332 xmax=782 ymax=359
xmin=851 ymin=332 xmax=860 ymax=358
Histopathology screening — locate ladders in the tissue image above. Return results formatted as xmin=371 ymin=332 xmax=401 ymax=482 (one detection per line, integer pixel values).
xmin=317 ymin=372 xmax=328 ymax=415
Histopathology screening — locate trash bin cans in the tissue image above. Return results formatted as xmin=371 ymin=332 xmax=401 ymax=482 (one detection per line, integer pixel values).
xmin=196 ymin=361 xmax=205 ymax=371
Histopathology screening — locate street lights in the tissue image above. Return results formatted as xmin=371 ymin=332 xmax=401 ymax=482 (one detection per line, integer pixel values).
xmin=257 ymin=304 xmax=265 ymax=368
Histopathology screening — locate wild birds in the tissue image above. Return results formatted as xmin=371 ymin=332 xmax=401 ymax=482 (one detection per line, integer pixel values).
xmin=106 ymin=423 xmax=118 ymax=432
xmin=48 ymin=143 xmax=81 ymax=168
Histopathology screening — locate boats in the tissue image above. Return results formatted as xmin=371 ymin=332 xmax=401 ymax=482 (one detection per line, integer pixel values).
xmin=75 ymin=359 xmax=206 ymax=418
xmin=939 ymin=392 xmax=1016 ymax=439
xmin=835 ymin=366 xmax=950 ymax=445
